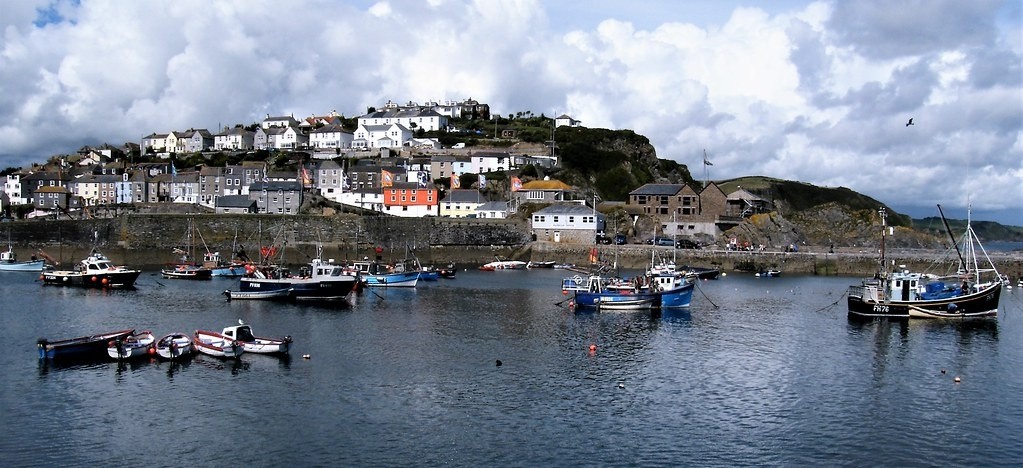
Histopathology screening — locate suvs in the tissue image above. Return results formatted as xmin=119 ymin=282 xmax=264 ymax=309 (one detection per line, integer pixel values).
xmin=599 ymin=234 xmax=698 ymax=249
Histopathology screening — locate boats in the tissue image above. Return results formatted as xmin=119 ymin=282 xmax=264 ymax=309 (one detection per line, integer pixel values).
xmin=678 ymin=264 xmax=721 ymax=280
xmin=642 ymin=208 xmax=695 ymax=310
xmin=907 ymin=304 xmax=999 ymax=320
xmin=36 ymin=328 xmax=135 ymax=360
xmin=153 ymin=333 xmax=193 ymax=360
xmin=191 ymin=329 xmax=246 ymax=358
xmin=560 ymin=191 xmax=663 ymax=310
xmin=478 ymin=254 xmax=526 ymax=269
xmin=105 ymin=329 xmax=157 ymax=360
xmin=848 ymin=195 xmax=1003 ymax=318
xmin=0 ymin=194 xmax=461 ymax=305
xmin=529 ymin=261 xmax=559 ymax=269
xmin=221 ymin=326 xmax=292 ymax=353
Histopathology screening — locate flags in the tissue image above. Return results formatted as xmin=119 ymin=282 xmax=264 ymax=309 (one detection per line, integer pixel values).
xmin=704 ymin=149 xmax=713 ymax=166
xmin=381 ymin=169 xmax=397 ymax=188
xmin=262 ymin=163 xmax=269 ymax=183
xmin=417 ymin=172 xmax=427 ymax=189
xmin=451 ymin=174 xmax=460 ymax=189
xmin=172 ymin=161 xmax=177 ymax=178
xmin=479 ymin=174 xmax=486 ymax=189
xmin=511 ymin=176 xmax=522 ymax=192
xmin=301 ymin=164 xmax=310 ymax=186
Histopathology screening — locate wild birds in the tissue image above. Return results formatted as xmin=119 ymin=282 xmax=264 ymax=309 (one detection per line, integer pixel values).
xmin=905 ymin=117 xmax=915 ymax=128
